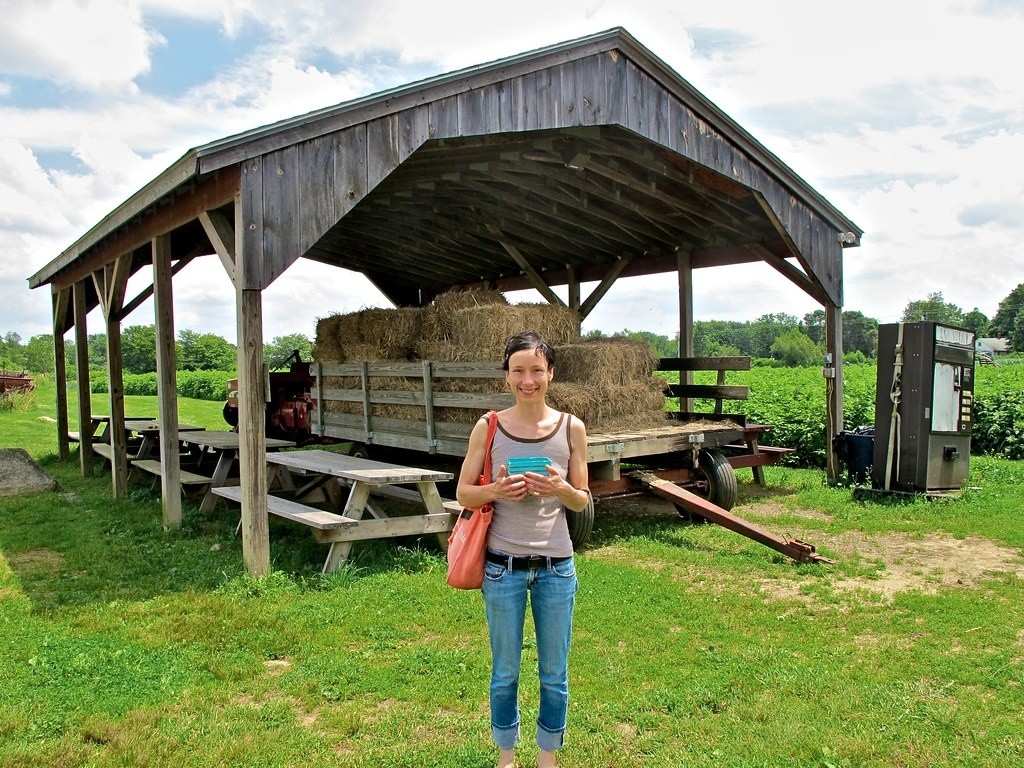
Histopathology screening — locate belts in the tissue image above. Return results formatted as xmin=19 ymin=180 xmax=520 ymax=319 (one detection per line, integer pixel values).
xmin=485 ymin=549 xmax=572 ymax=570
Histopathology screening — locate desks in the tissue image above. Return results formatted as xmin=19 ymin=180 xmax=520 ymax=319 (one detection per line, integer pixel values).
xmin=66 ymin=415 xmax=456 ymax=578
xmin=717 ymin=422 xmax=799 ymax=486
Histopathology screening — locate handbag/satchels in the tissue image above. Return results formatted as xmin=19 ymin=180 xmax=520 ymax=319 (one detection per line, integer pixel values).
xmin=447 ymin=472 xmax=493 ymax=590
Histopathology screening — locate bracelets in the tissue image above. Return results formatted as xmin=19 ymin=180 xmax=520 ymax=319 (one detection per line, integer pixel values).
xmin=575 ymin=488 xmax=589 ymax=494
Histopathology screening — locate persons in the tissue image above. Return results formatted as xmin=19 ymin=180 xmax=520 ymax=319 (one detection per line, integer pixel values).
xmin=455 ymin=329 xmax=591 ymax=768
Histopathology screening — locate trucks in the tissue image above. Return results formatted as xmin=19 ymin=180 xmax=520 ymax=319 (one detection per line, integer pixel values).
xmin=222 ymin=349 xmax=755 ymax=550
